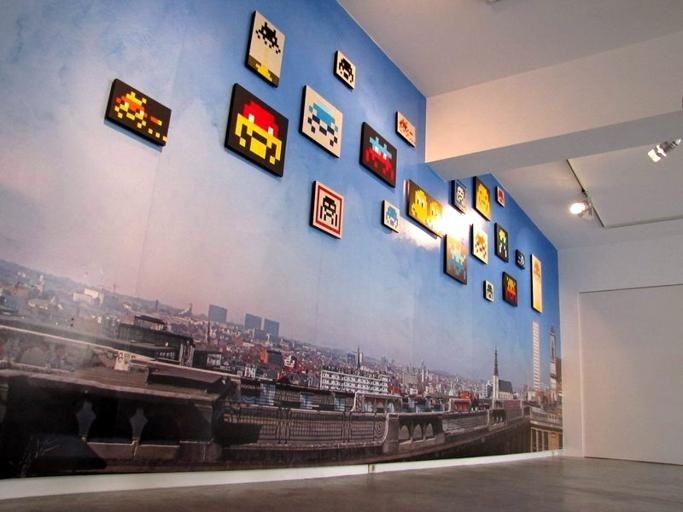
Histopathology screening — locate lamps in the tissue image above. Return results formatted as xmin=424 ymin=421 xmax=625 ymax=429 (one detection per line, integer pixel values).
xmin=569 ymin=199 xmax=594 ymax=219
xmin=647 ymin=138 xmax=681 ymax=165
